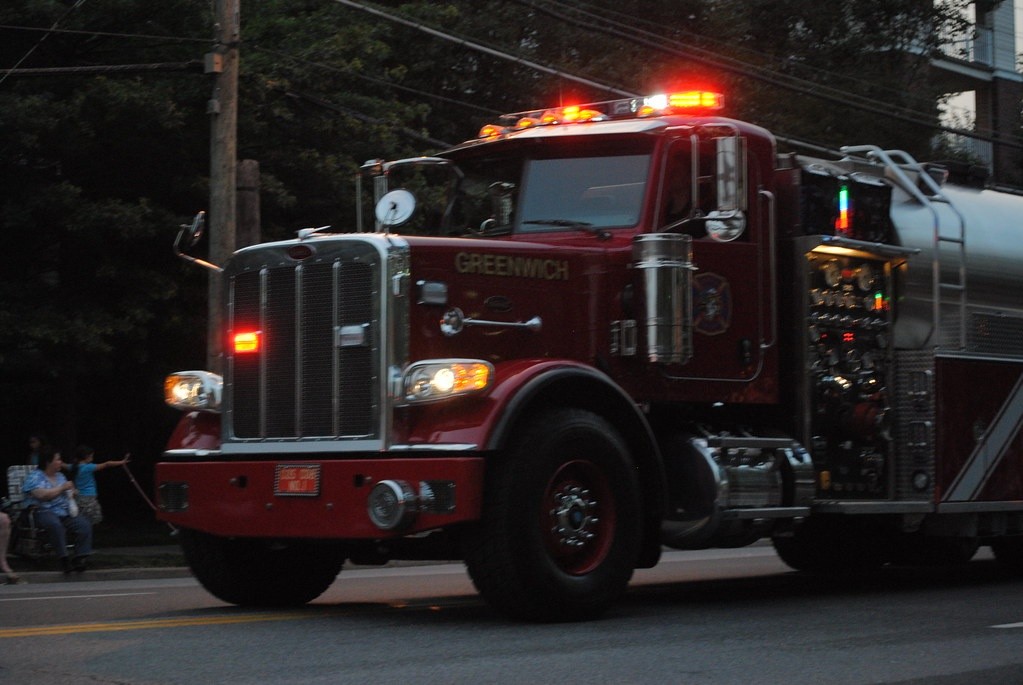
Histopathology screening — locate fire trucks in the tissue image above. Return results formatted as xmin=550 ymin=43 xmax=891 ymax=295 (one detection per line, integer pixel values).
xmin=152 ymin=77 xmax=1022 ymax=631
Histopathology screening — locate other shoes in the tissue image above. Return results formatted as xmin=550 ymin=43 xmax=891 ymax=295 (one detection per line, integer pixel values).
xmin=75 ymin=560 xmax=86 ymax=571
xmin=63 ymin=561 xmax=73 ymax=571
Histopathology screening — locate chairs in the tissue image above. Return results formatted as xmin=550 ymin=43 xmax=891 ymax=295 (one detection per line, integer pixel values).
xmin=1 ymin=464 xmax=77 ymax=560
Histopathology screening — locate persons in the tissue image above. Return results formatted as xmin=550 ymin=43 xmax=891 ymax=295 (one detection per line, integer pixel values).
xmin=27 ymin=435 xmax=43 ymax=467
xmin=21 ymin=450 xmax=92 ymax=574
xmin=46 ymin=444 xmax=132 ymax=548
xmin=660 ymin=149 xmax=718 ymax=238
xmin=0 ymin=511 xmax=22 ymax=582
